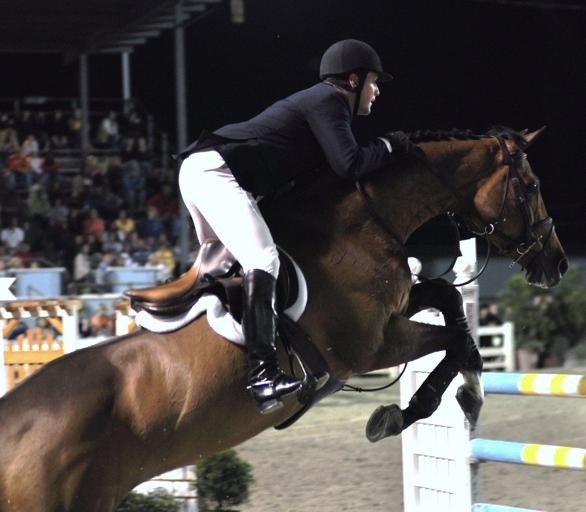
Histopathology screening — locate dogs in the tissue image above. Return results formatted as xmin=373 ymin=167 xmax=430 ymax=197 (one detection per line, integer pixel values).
xmin=0 ymin=120 xmax=569 ymax=512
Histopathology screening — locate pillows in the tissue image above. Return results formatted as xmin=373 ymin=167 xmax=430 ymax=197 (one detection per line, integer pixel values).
xmin=320 ymin=38 xmax=394 ymax=81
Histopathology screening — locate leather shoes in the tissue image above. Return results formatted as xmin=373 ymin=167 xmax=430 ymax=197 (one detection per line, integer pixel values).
xmin=381 ymin=130 xmax=409 ymax=154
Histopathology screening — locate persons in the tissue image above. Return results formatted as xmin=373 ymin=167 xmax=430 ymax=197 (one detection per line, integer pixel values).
xmin=0 ymin=98 xmax=200 ymax=288
xmin=172 ymin=38 xmax=408 ymax=414
xmin=479 ymin=303 xmax=505 ymax=371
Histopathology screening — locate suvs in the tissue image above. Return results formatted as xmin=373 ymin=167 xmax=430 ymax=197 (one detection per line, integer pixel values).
xmin=106 ymin=267 xmax=166 ymax=300
xmin=12 ymin=265 xmax=67 ymax=336
xmin=79 ymin=295 xmax=122 ymax=338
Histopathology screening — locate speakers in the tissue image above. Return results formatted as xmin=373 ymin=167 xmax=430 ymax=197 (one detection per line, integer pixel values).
xmin=241 ymin=269 xmax=330 ymax=416
